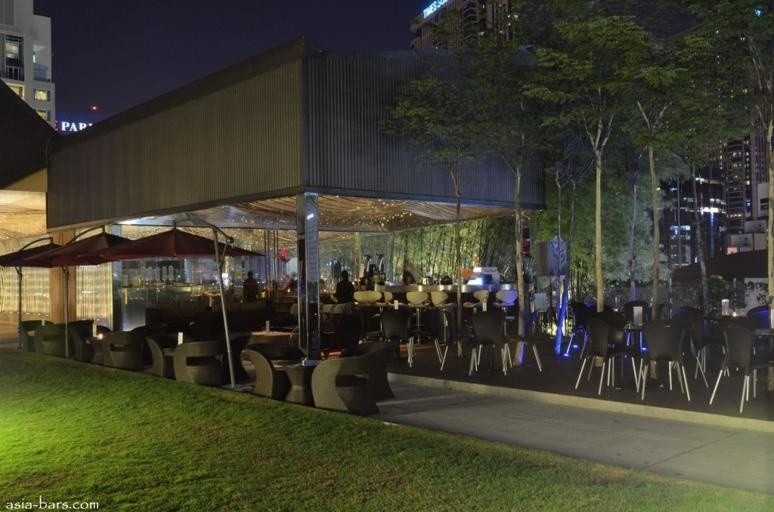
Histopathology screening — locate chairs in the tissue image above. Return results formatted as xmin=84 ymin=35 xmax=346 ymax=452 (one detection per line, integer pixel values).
xmin=353 ymin=286 xmax=548 ymax=375
xmin=563 ymin=299 xmax=774 ymax=414
xmin=17 ymin=318 xmax=395 ymax=416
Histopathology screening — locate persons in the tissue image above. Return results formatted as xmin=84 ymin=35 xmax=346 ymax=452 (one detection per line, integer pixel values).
xmin=333 ymin=270 xmax=355 ymax=302
xmin=243 ymin=271 xmax=258 ymax=303
